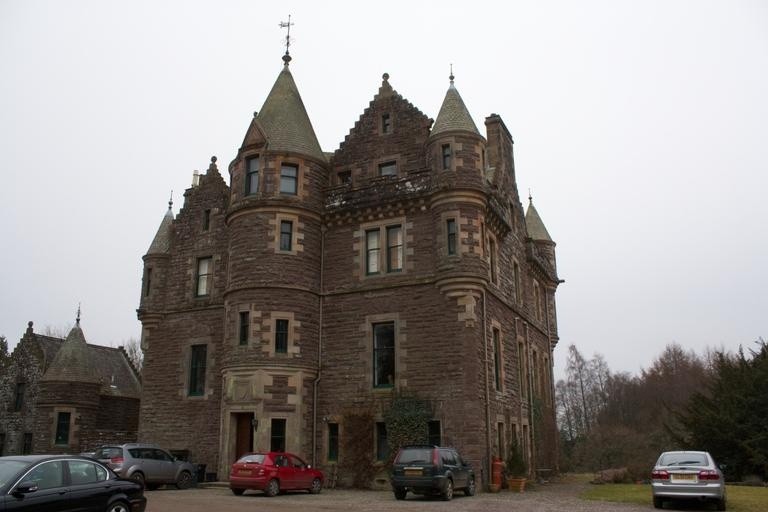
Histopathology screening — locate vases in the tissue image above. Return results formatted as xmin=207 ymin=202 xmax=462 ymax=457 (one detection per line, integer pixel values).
xmin=537 ymin=469 xmax=552 ymax=485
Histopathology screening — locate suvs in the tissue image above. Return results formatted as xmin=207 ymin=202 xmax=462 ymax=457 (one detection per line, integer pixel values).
xmin=97 ymin=441 xmax=195 ymax=489
xmin=388 ymin=443 xmax=477 ymax=500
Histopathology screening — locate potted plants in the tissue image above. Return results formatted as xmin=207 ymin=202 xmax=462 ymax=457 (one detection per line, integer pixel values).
xmin=507 ymin=441 xmax=527 ymax=493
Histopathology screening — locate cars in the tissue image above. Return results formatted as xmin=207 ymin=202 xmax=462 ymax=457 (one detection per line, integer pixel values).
xmin=0 ymin=452 xmax=148 ymax=512
xmin=227 ymin=452 xmax=324 ymax=497
xmin=650 ymin=449 xmax=727 ymax=508
